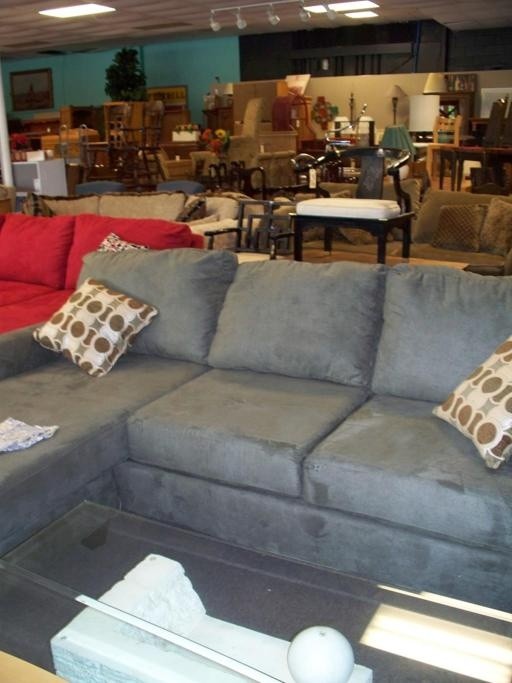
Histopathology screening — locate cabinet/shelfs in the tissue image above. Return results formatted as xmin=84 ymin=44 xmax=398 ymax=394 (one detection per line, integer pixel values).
xmin=291 ymin=96 xmax=313 ymax=151
xmin=11 ymin=157 xmax=68 ymax=197
xmin=422 ymin=90 xmax=474 ymax=135
xmin=160 ymin=143 xmax=200 ymax=160
xmin=232 ymin=80 xmax=288 ymax=136
xmin=214 ymin=107 xmax=233 ymax=136
xmin=40 ymin=127 xmax=101 ymax=159
xmin=103 ymin=101 xmax=144 ymax=147
xmin=301 ymin=139 xmax=326 ymax=165
xmin=470 ymin=118 xmax=507 ymax=131
xmin=22 ymin=118 xmax=60 ymax=143
xmin=229 ymin=133 xmax=298 ymax=186
xmin=58 ymin=105 xmax=97 ymax=131
xmin=202 ymin=109 xmax=217 ymax=130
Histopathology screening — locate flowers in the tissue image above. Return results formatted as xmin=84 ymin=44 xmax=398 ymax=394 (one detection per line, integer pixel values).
xmin=196 ymin=127 xmax=231 ymax=156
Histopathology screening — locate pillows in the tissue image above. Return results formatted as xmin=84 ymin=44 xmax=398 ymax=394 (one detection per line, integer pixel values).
xmin=0 ymin=213 xmax=76 ymax=289
xmin=208 ymin=260 xmax=392 ymax=389
xmin=32 ymin=276 xmax=158 ymax=378
xmin=64 ymin=213 xmax=193 ymax=290
xmin=320 ymin=189 xmax=376 ymax=245
xmin=22 ymin=191 xmax=58 ymax=218
xmin=176 ymin=214 xmax=220 ymax=227
xmin=414 ymin=189 xmax=512 ymax=243
xmin=432 ymin=335 xmax=512 ymax=470
xmin=76 ymin=247 xmax=239 ymax=366
xmin=318 ymin=182 xmax=358 ymax=198
xmin=370 ymin=263 xmax=512 ymax=403
xmin=430 ymin=204 xmax=488 ymax=252
xmin=478 ymin=197 xmax=512 ymax=257
xmin=97 ymin=231 xmax=150 ymax=253
xmin=383 ymin=178 xmax=420 ymax=241
xmin=172 ymin=196 xmax=207 ymax=223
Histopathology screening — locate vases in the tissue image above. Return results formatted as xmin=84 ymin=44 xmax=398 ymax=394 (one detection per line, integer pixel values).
xmin=211 ymin=158 xmax=228 ymax=178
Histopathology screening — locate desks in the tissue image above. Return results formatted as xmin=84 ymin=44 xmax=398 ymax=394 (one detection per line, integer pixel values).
xmin=439 ymin=145 xmax=512 ymax=192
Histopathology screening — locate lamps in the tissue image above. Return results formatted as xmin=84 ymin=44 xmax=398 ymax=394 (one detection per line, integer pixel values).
xmin=296 ymin=1 xmax=311 ymax=23
xmin=294 ymin=149 xmax=347 ymax=180
xmin=378 ymin=124 xmax=417 ymax=181
xmin=208 ymin=12 xmax=222 ymax=32
xmin=382 ymin=84 xmax=407 ymax=125
xmin=231 ymin=8 xmax=248 ymax=29
xmin=321 ymin=1 xmax=337 ymax=21
xmin=265 ymin=6 xmax=281 ymax=26
xmin=221 ymin=83 xmax=234 ymax=107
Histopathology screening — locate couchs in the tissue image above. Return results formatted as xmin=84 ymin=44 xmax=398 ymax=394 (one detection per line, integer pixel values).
xmin=0 ymin=321 xmax=512 ymax=614
xmin=258 ymin=206 xmax=512 ymax=275
xmin=0 ymin=234 xmax=205 ymax=336
xmin=157 ymin=149 xmax=192 ymax=181
xmin=18 ymin=192 xmax=264 ymax=249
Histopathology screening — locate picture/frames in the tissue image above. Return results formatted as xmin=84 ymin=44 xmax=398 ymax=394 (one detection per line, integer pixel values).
xmin=9 ymin=67 xmax=55 ymax=111
xmin=146 ymin=85 xmax=188 ymax=109
xmin=445 ymin=73 xmax=477 ymax=93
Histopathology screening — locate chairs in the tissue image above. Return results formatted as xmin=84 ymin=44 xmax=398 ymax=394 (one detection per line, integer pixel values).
xmin=74 ymin=180 xmax=127 ymax=198
xmin=230 ymin=160 xmax=246 ymax=169
xmin=208 ymin=163 xmax=227 ymax=192
xmin=409 ymin=114 xmax=463 ymax=180
xmin=229 ymin=166 xmax=267 ymax=202
xmin=204 ymin=200 xmax=297 ymax=260
xmin=157 ymin=179 xmax=204 ymax=195
xmin=111 ymin=100 xmax=166 ymax=188
